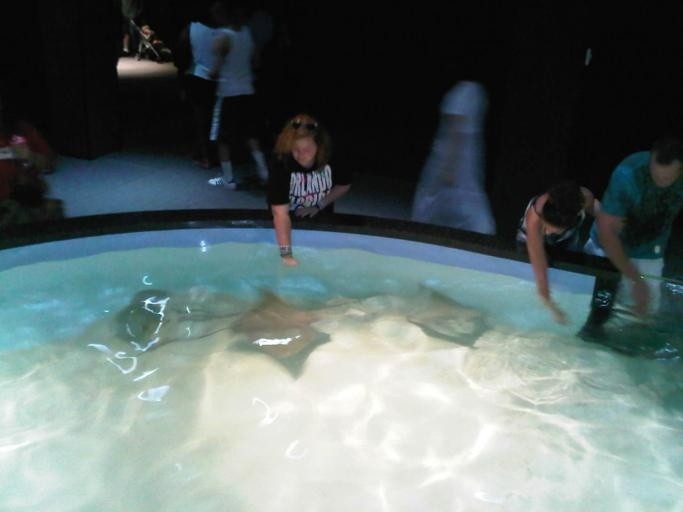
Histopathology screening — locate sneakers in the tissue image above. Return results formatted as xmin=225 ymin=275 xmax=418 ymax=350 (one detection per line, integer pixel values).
xmin=208 ymin=177 xmax=236 ymax=189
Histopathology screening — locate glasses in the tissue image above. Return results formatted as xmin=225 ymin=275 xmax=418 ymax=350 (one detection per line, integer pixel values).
xmin=291 ymin=122 xmax=316 ymax=130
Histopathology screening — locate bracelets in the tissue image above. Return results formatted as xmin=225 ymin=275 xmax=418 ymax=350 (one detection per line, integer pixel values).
xmin=279 ymin=246 xmax=291 ymax=257
xmin=315 ymin=204 xmax=321 ymax=210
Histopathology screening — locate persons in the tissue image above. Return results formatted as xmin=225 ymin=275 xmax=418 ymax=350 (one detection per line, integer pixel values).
xmin=193 ymin=1 xmax=274 ymax=189
xmin=263 ymin=110 xmax=354 ymax=264
xmin=409 ymin=77 xmax=498 ymax=235
xmin=119 ymin=1 xmax=142 ymax=54
xmin=582 ymin=139 xmax=682 ymax=311
xmin=141 ymin=23 xmax=171 ymax=54
xmin=0 ymin=99 xmax=64 ymax=228
xmin=514 ymin=174 xmax=654 ymax=326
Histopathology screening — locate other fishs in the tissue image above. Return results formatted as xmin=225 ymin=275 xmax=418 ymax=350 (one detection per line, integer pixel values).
xmin=113 ymin=287 xmax=496 ymax=383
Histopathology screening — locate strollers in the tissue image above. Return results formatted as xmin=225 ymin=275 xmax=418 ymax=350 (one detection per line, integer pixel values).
xmin=129 ymin=15 xmax=172 ymax=63
